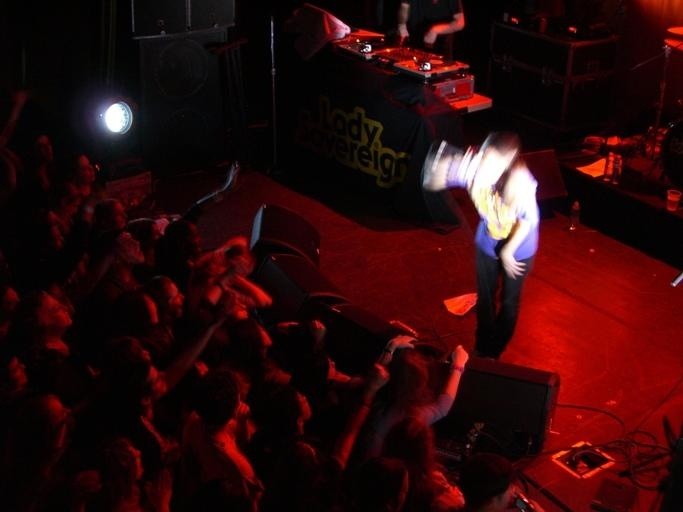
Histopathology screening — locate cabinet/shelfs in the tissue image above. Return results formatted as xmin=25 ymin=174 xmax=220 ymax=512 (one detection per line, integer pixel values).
xmin=491 ymin=21 xmax=623 ymax=136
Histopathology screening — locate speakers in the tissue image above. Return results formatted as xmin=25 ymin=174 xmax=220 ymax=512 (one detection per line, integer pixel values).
xmin=188 ymin=0 xmax=235 ymax=34
xmin=266 ymin=251 xmax=346 ymax=319
xmin=517 ymin=148 xmax=569 ymax=215
xmin=437 ymin=351 xmax=559 ymax=462
xmin=325 ymin=303 xmax=392 ymax=367
xmin=250 ymin=202 xmax=320 ymax=265
xmin=137 ymin=36 xmax=225 ymax=175
xmin=131 ymin=0 xmax=188 ymax=39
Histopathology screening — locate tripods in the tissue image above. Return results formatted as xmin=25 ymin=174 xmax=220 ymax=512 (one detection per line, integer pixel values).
xmin=645 ymin=46 xmax=678 ymax=197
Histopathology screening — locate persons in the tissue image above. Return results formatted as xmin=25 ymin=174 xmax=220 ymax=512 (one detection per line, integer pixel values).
xmin=0 ymin=91 xmax=468 ymax=512
xmin=395 ymin=0 xmax=465 ymax=59
xmin=422 ymin=127 xmax=541 ymax=360
